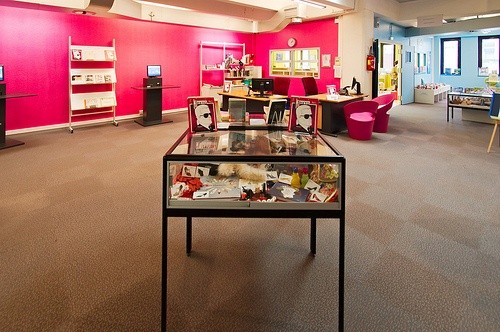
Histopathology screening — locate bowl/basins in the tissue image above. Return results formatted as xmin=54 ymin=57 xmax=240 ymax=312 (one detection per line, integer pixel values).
xmin=233 ymin=80 xmax=242 ymax=84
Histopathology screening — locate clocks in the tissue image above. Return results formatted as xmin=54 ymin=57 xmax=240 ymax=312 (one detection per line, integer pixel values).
xmin=287 ymin=37 xmax=298 ymax=48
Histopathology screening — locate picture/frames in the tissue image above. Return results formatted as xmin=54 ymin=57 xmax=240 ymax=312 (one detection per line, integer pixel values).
xmin=266 ymin=99 xmax=287 ymax=125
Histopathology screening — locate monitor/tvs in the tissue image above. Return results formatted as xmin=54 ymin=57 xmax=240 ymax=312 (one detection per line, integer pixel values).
xmin=351 ymin=77 xmax=361 ymax=95
xmin=147 ymin=65 xmax=161 ymax=78
xmin=252 ymin=78 xmax=274 ymax=98
xmin=0 ymin=66 xmax=4 ymax=80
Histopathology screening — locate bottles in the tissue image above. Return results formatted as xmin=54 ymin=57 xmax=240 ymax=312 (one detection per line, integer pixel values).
xmin=291 ymin=167 xmax=300 ymax=187
xmin=302 ymin=168 xmax=309 ymax=187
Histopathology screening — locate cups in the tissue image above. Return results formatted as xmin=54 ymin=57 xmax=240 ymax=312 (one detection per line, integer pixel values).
xmin=229 ymin=70 xmax=245 ymax=77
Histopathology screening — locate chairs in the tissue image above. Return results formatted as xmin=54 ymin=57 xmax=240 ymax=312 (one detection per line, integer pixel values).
xmin=273 ymin=77 xmax=290 ymax=96
xmin=372 ymin=94 xmax=394 ymax=133
xmin=486 ymin=92 xmax=500 ymax=153
xmin=302 ymin=77 xmax=318 ymax=96
xmin=343 ymin=100 xmax=379 ymax=140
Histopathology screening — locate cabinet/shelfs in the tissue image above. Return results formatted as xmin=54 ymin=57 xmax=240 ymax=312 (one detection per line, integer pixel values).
xmin=199 ymin=40 xmax=262 ymax=108
xmin=67 ymin=37 xmax=119 ymax=134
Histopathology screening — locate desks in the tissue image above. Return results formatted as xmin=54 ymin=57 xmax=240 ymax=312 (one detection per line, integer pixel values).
xmin=307 ymin=91 xmax=369 ymax=138
xmin=131 ymin=85 xmax=181 ymax=127
xmin=162 ymin=114 xmax=347 ymax=332
xmin=415 ymin=85 xmax=450 ymax=104
xmin=446 ymin=92 xmax=492 ymax=122
xmin=216 ymin=89 xmax=288 ymax=113
